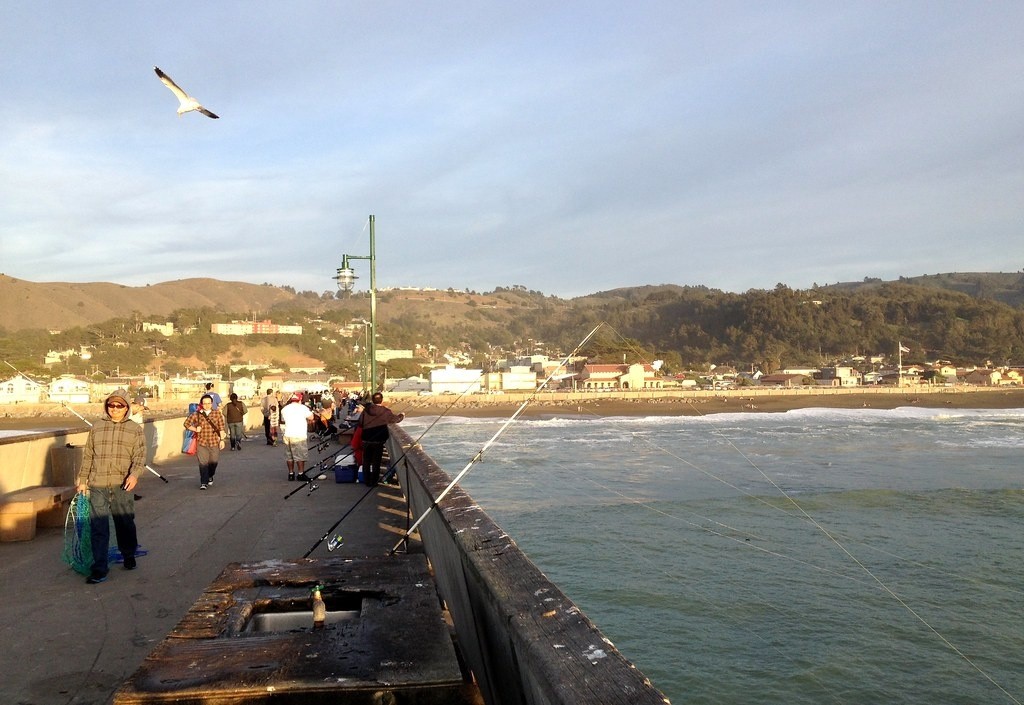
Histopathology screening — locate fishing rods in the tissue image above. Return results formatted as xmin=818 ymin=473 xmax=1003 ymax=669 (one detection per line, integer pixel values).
xmin=388 ymin=315 xmax=604 ymax=557
xmin=302 ymin=348 xmax=511 ymax=561
xmin=0 ymin=358 xmax=169 ymax=484
xmin=281 ymin=388 xmax=436 ymax=499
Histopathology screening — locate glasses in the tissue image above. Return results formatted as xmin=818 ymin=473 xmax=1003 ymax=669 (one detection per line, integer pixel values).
xmin=107 ymin=403 xmax=125 ymax=408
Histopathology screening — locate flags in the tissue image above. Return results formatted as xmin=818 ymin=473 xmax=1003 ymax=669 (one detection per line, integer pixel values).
xmin=901 ymin=345 xmax=911 ymax=353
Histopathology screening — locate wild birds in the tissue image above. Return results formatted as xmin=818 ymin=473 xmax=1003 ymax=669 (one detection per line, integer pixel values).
xmin=152 ymin=66 xmax=220 ymax=120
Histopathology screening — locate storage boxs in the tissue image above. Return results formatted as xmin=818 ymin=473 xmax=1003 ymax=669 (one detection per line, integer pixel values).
xmin=358 ymin=465 xmax=373 ymax=482
xmin=335 ymin=454 xmax=358 ymax=483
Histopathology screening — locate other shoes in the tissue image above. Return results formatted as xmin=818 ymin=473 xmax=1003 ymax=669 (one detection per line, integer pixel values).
xmin=86 ymin=567 xmax=110 ymax=582
xmin=235 ymin=441 xmax=241 ymax=450
xmin=288 ymin=472 xmax=295 ymax=481
xmin=123 ymin=555 xmax=136 ymax=569
xmin=208 ymin=477 xmax=213 ymax=485
xmin=296 ymin=472 xmax=312 ymax=481
xmin=231 ymin=447 xmax=235 ymax=451
xmin=200 ymin=484 xmax=208 ymax=490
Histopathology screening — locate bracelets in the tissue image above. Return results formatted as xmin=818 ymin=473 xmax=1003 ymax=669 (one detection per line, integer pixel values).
xmin=220 ymin=439 xmax=225 ymax=441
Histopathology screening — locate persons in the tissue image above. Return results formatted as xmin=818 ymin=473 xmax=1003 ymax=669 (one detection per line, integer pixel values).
xmin=184 ymin=394 xmax=226 ymax=490
xmin=261 ymin=386 xmax=375 ymax=446
xmin=281 ymin=392 xmax=315 ymax=482
xmin=350 ymin=401 xmax=373 ymax=483
xmin=205 ymin=383 xmax=221 ymax=411
xmin=358 ymin=393 xmax=404 ymax=488
xmin=75 ymin=389 xmax=148 ymax=584
xmin=223 ymin=393 xmax=247 ymax=451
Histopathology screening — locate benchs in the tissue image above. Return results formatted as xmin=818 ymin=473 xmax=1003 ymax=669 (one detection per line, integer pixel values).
xmin=337 ymin=429 xmax=355 ymax=445
xmin=0 ymin=486 xmax=78 ymax=542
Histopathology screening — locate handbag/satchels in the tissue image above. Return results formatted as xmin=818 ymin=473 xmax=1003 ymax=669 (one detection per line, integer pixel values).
xmin=181 ymin=429 xmax=197 ymax=454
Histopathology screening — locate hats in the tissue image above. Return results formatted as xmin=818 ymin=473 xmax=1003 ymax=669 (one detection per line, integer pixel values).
xmin=291 ymin=393 xmax=302 ymax=401
xmin=107 ymin=396 xmax=128 ymax=405
